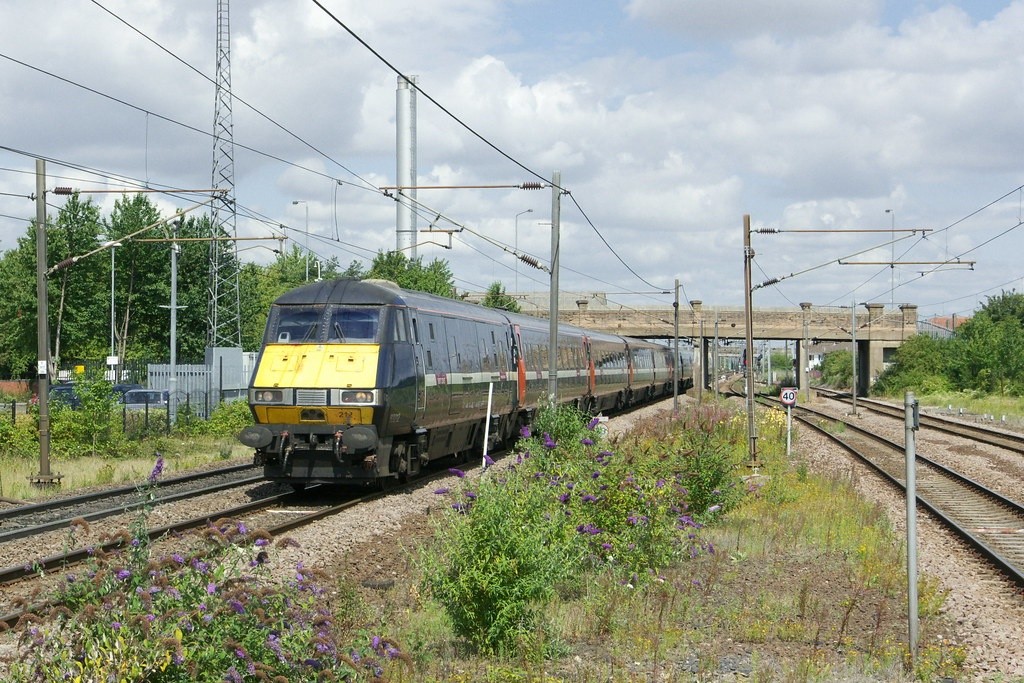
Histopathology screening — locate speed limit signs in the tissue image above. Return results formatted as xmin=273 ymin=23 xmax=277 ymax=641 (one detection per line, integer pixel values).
xmin=780 ymin=389 xmax=796 ymax=404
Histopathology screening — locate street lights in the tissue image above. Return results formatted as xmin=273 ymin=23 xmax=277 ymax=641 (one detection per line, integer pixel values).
xmin=104 ymin=241 xmax=122 ymax=383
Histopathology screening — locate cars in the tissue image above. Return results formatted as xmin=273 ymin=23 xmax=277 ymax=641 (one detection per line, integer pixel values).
xmin=118 ymin=388 xmax=181 ymax=410
xmin=109 ymin=382 xmax=144 ymax=397
xmin=48 ymin=380 xmax=95 ymax=412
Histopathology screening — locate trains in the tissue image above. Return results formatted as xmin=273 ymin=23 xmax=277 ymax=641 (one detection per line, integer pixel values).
xmin=237 ymin=278 xmax=764 ymax=493
